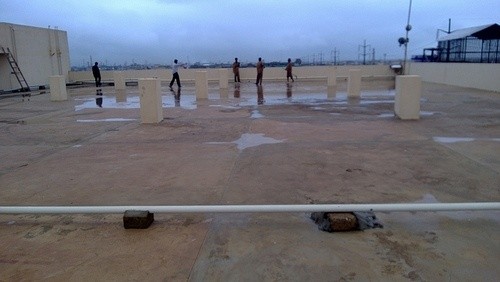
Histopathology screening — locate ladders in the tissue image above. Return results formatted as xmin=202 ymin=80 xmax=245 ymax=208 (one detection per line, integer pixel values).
xmin=1 ymin=46 xmax=31 ymax=92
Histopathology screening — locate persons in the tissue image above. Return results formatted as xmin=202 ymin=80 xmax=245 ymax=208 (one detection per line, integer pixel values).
xmin=92 ymin=62 xmax=102 ymax=86
xmin=233 ymin=57 xmax=240 ymax=82
xmin=254 ymin=57 xmax=265 ymax=85
xmin=169 ymin=59 xmax=187 ymax=88
xmin=284 ymin=58 xmax=295 ymax=82
